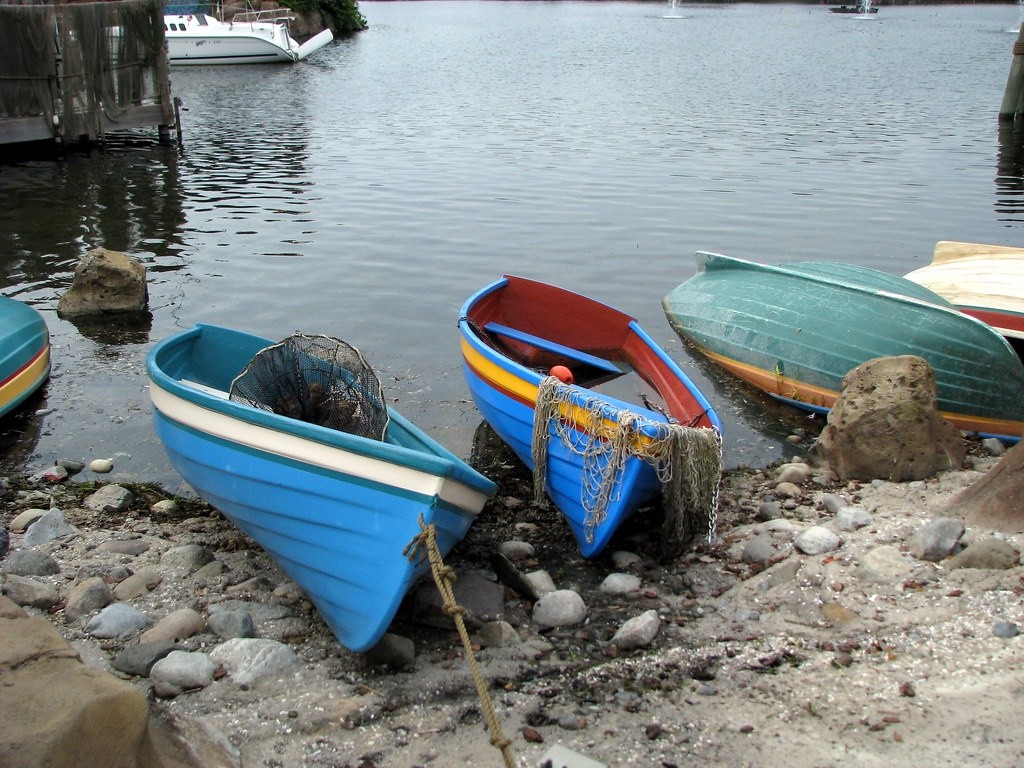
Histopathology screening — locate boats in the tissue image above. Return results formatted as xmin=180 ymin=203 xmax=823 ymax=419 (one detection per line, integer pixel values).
xmin=457 ymin=274 xmax=725 ymax=558
xmin=903 ymin=242 xmax=1024 ymax=358
xmin=146 ymin=322 xmax=499 ymax=653
xmin=662 ymin=250 xmax=1024 ymax=447
xmin=829 ymin=4 xmax=878 ymax=13
xmin=69 ymin=8 xmax=334 ymax=65
xmin=0 ymin=295 xmax=52 ymax=416
